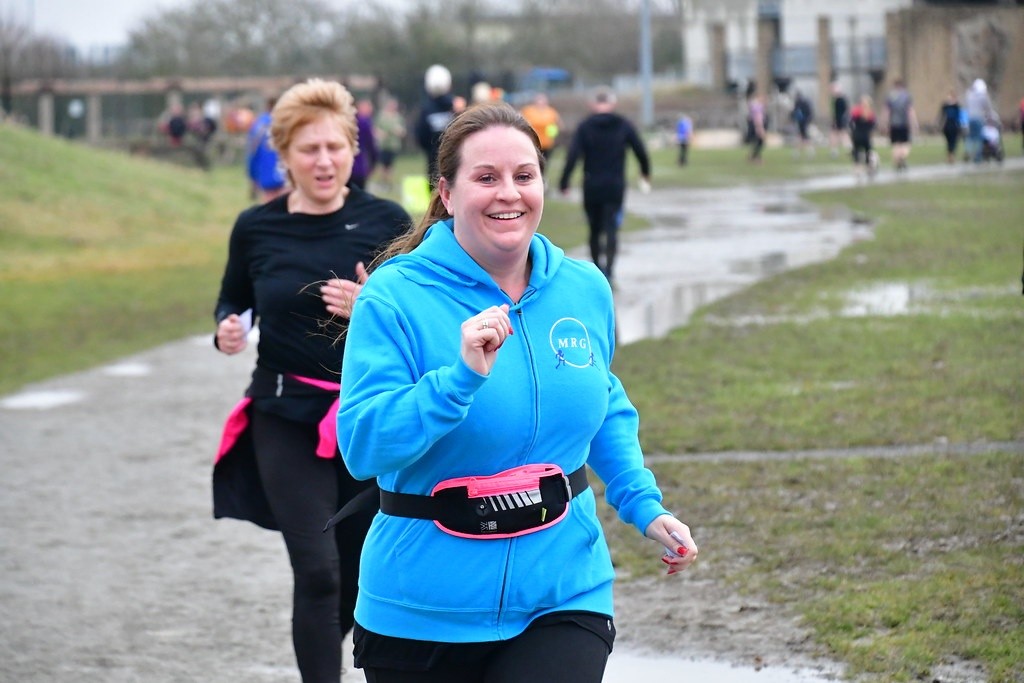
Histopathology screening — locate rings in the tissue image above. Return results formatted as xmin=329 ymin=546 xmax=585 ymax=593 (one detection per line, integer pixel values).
xmin=482 ymin=321 xmax=489 ymax=330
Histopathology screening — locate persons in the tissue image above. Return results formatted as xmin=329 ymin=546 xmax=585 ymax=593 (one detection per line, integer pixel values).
xmin=210 ymin=78 xmax=418 ymax=682
xmin=559 ymin=84 xmax=656 ymax=294
xmin=336 ymin=100 xmax=699 ymax=683
xmin=152 ymin=61 xmax=1006 ymax=205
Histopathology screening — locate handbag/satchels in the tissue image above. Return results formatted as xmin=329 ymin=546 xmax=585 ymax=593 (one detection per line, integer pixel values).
xmin=375 ymin=462 xmax=593 ymax=541
